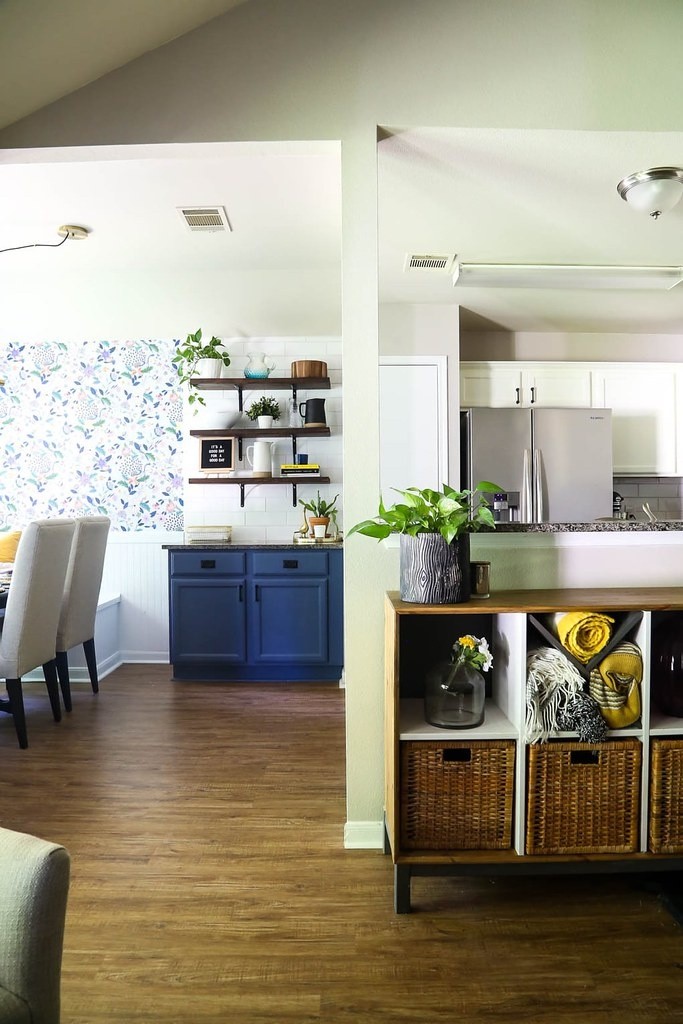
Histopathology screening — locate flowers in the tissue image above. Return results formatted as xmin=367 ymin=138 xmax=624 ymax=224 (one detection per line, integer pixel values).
xmin=453 ymin=634 xmax=493 ymax=671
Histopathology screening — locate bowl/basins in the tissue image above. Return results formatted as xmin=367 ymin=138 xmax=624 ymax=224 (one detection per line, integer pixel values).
xmin=290 ymin=360 xmax=327 ymax=378
xmin=197 ymin=412 xmax=243 ymax=428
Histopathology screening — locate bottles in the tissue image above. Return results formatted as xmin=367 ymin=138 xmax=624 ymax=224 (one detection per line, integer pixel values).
xmin=288 ymin=399 xmax=300 ymax=427
xmin=614 ymin=502 xmax=630 ymax=519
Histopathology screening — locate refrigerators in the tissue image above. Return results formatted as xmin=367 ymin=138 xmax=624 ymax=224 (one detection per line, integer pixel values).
xmin=461 ymin=407 xmax=614 ymax=523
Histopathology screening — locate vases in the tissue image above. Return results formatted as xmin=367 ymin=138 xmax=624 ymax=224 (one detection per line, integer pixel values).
xmin=423 ymin=658 xmax=485 ymax=730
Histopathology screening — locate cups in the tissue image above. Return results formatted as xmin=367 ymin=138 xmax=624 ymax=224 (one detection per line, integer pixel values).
xmin=470 ymin=561 xmax=491 ymax=599
xmin=294 ymin=454 xmax=308 ymax=465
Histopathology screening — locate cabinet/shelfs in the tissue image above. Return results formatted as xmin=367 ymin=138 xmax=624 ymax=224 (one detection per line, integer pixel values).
xmin=188 ymin=377 xmax=330 ymax=484
xmin=461 ymin=363 xmax=683 ymax=480
xmin=379 ymin=588 xmax=683 ymax=913
xmin=167 ymin=550 xmax=343 ymax=683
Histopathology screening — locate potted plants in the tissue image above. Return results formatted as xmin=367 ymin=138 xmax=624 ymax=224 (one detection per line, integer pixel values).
xmin=346 ymin=481 xmax=507 ymax=605
xmin=294 ymin=490 xmax=340 ymax=535
xmin=244 ymin=396 xmax=281 ymax=428
xmin=171 ymin=328 xmax=230 ymax=407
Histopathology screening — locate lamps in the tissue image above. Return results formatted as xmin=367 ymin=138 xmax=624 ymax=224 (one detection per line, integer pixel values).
xmin=617 ymin=166 xmax=683 ymax=220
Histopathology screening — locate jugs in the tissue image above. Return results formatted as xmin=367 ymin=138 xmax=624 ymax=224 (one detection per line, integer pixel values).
xmin=246 ymin=441 xmax=274 ymax=477
xmin=244 ymin=352 xmax=276 ymax=379
xmin=299 ymin=399 xmax=326 ymax=427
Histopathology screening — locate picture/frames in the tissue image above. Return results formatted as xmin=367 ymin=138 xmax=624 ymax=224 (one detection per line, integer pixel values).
xmin=198 ymin=436 xmax=234 ymax=474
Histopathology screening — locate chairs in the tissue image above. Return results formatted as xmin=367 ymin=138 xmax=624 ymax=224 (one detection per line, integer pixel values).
xmin=0 ymin=519 xmax=77 ymax=749
xmin=0 ymin=824 xmax=70 ymax=1024
xmin=55 ymin=517 xmax=111 ymax=711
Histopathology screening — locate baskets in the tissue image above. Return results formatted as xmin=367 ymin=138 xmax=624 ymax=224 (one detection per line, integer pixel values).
xmin=525 ymin=739 xmax=642 ymax=854
xmin=650 ymin=739 xmax=683 ymax=855
xmin=396 ymin=741 xmax=514 ymax=852
